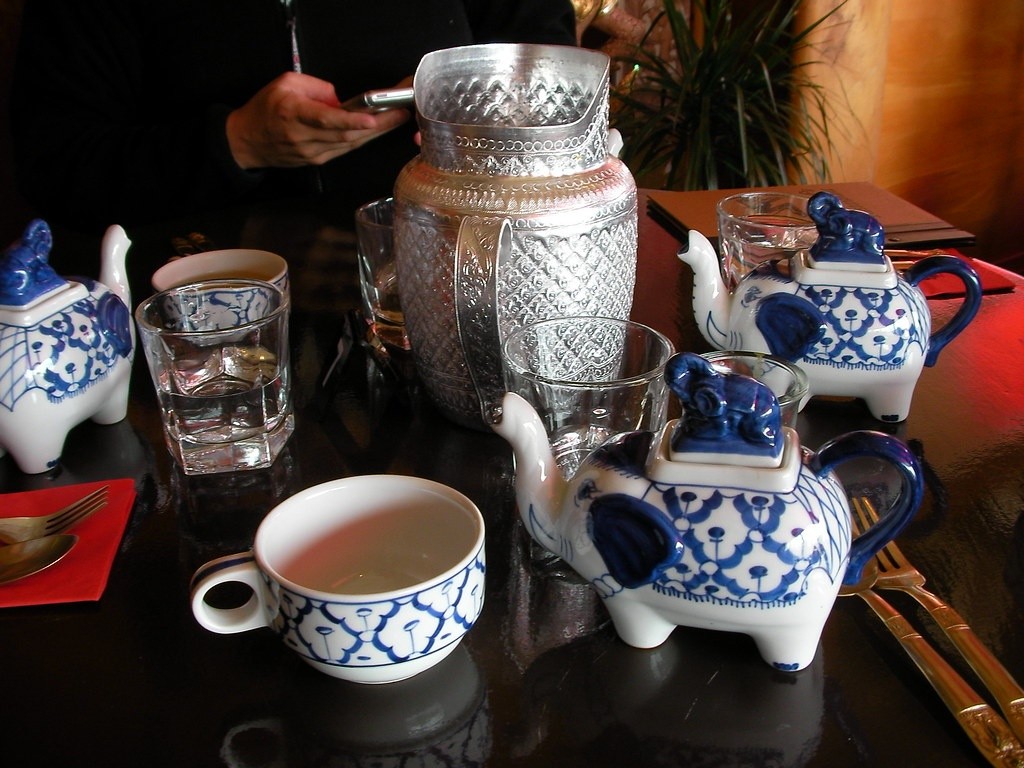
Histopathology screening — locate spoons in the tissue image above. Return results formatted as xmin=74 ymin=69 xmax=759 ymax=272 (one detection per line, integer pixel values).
xmin=0 ymin=533 xmax=79 ymax=585
xmin=837 ymin=531 xmax=1024 ymax=768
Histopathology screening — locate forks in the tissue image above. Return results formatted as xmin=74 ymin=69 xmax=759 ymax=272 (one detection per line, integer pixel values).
xmin=848 ymin=496 xmax=1024 ymax=746
xmin=0 ymin=485 xmax=108 ymax=544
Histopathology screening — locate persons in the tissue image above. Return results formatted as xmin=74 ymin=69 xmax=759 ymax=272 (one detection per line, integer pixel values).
xmin=1 ymin=1 xmax=582 ymax=274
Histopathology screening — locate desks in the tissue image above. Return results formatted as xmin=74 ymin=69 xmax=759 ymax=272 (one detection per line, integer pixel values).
xmin=0 ymin=186 xmax=1024 ymax=768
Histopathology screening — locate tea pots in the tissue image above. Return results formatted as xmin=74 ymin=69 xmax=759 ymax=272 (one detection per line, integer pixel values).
xmin=677 ymin=191 xmax=983 ymax=422
xmin=487 ymin=351 xmax=928 ymax=675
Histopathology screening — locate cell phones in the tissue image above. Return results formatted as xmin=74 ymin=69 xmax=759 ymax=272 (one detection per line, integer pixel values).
xmin=340 ymin=87 xmax=414 ymax=114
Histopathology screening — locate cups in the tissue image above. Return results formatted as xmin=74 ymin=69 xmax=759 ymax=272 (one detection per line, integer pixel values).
xmin=501 ymin=315 xmax=676 ymax=574
xmin=355 ymin=196 xmax=410 ymax=352
xmin=135 ymin=278 xmax=295 ymax=476
xmin=716 ymin=190 xmax=822 ymax=294
xmin=680 ymin=350 xmax=809 ymax=431
xmin=219 ymin=633 xmax=492 ymax=768
xmin=152 ymin=249 xmax=291 ymax=320
xmin=191 ymin=473 xmax=486 ymax=685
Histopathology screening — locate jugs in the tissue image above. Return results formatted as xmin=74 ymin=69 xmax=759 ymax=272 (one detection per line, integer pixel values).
xmin=393 ymin=43 xmax=639 ymax=432
